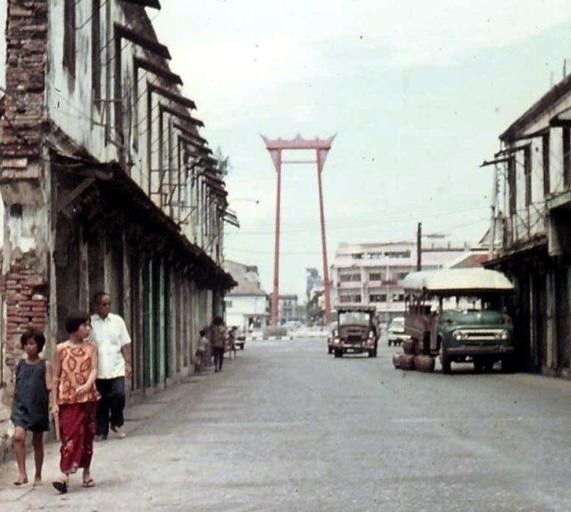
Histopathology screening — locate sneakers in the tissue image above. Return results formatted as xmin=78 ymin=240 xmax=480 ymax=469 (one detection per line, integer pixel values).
xmin=109 ymin=424 xmax=127 ymax=438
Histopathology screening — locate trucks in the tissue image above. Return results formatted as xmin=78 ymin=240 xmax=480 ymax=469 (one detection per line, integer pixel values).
xmin=333 ymin=302 xmax=378 ymax=360
xmin=404 ymin=267 xmax=516 ymax=373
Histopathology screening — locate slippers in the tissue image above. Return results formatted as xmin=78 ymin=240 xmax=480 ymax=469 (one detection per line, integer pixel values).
xmin=81 ymin=478 xmax=95 ymax=487
xmin=52 ymin=477 xmax=72 ymax=493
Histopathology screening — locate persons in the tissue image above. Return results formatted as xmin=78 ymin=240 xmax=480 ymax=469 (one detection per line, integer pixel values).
xmin=51 ymin=311 xmax=102 ymax=492
xmin=10 ymin=329 xmax=54 ymax=489
xmin=194 ymin=317 xmax=239 ymax=375
xmin=82 ymin=291 xmax=133 ymax=442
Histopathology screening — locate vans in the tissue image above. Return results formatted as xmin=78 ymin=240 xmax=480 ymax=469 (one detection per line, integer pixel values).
xmin=387 ymin=317 xmax=412 ymax=348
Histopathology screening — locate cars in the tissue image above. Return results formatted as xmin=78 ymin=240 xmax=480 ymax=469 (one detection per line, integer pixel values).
xmin=328 ymin=330 xmax=339 ymax=352
xmin=226 ymin=326 xmax=247 ymax=350
xmin=282 ymin=321 xmax=302 ymax=330
